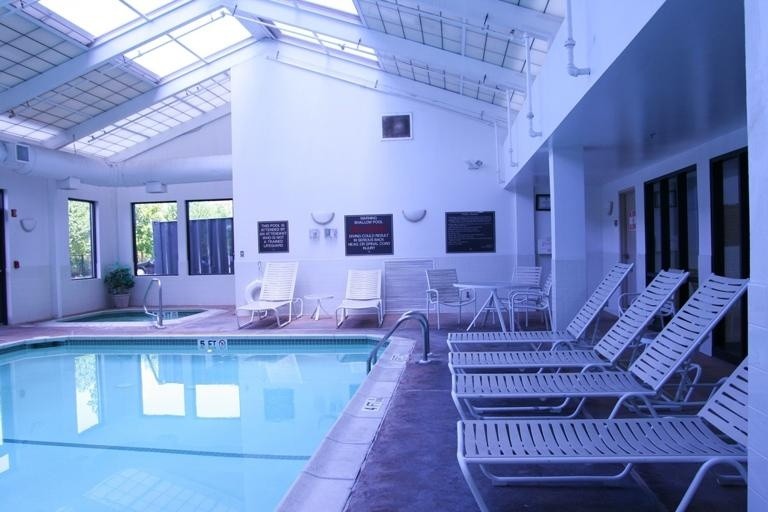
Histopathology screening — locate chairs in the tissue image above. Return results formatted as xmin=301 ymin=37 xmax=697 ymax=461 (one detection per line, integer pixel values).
xmin=508 ymin=273 xmax=552 ymax=332
xmin=457 ymin=359 xmax=748 ymax=512
xmin=423 ymin=269 xmax=477 ymax=331
xmin=451 ymin=273 xmax=749 ymax=485
xmin=234 ymin=262 xmax=303 ymax=328
xmin=499 ymin=266 xmax=542 ymax=326
xmin=447 ymin=261 xmax=635 ymax=403
xmin=447 ymin=270 xmax=690 ymax=420
xmin=334 ymin=269 xmax=384 ymax=328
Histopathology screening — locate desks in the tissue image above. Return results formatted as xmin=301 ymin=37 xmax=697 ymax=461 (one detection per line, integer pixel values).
xmin=304 ymin=294 xmax=333 ymax=321
xmin=453 ymin=281 xmax=537 ymax=332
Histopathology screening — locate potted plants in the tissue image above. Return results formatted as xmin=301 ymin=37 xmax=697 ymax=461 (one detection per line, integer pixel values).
xmin=104 ymin=268 xmax=135 ymax=308
